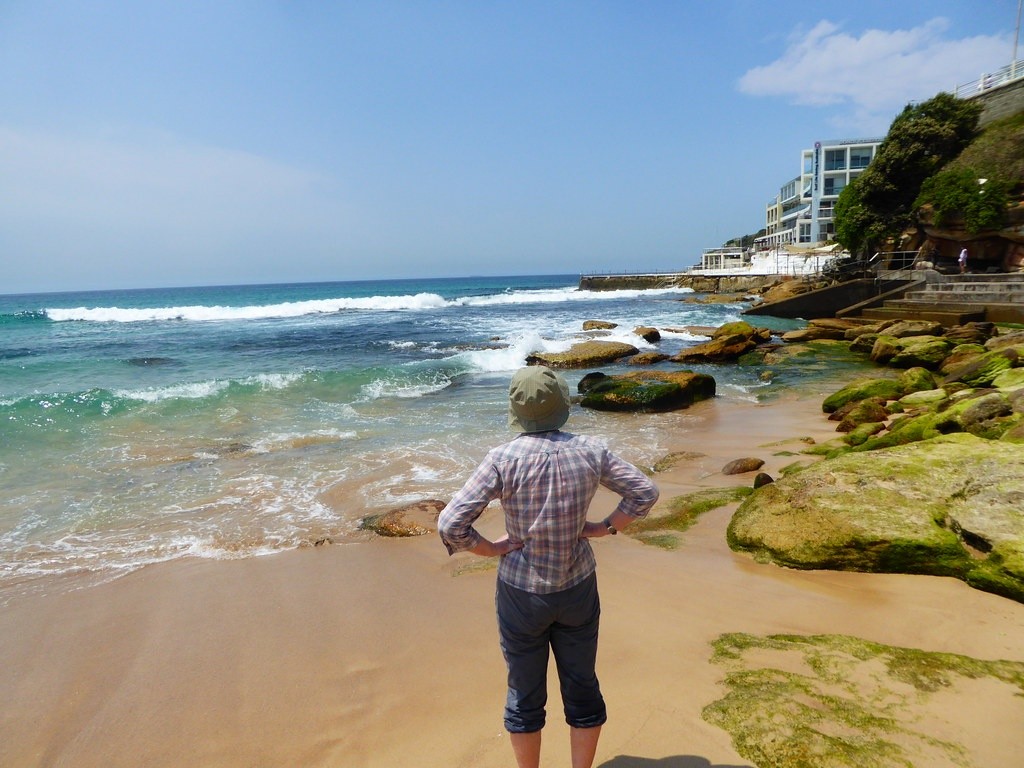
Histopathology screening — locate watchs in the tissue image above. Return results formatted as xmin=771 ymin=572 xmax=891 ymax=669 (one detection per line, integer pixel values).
xmin=603 ymin=517 xmax=617 ymax=535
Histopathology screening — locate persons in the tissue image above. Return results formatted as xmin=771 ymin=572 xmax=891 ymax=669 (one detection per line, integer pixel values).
xmin=958 ymin=245 xmax=967 ymax=274
xmin=437 ymin=365 xmax=659 ymax=768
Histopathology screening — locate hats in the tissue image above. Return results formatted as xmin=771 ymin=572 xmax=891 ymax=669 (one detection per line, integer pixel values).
xmin=508 ymin=365 xmax=570 ymax=433
